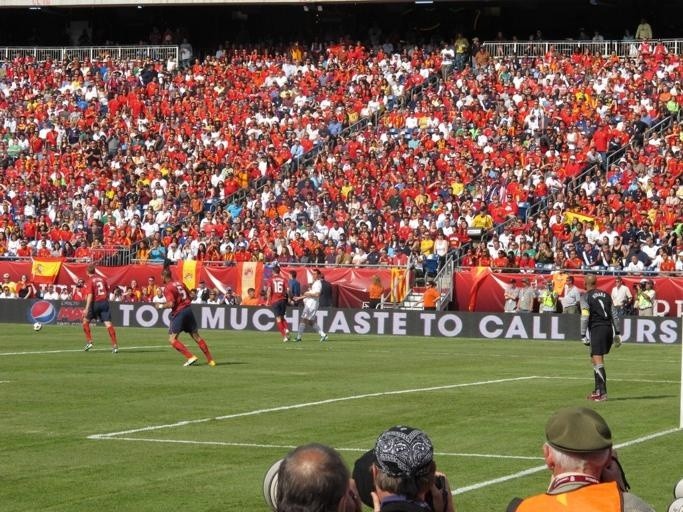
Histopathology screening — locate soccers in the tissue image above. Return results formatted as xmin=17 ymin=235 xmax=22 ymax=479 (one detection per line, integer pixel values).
xmin=33 ymin=322 xmax=42 ymax=331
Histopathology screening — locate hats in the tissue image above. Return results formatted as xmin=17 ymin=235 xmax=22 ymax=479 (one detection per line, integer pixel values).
xmin=639 ymin=209 xmax=650 ymax=217
xmin=543 ymin=406 xmax=613 ymax=454
xmin=508 ymin=277 xmax=517 ymax=284
xmin=225 ymin=286 xmax=233 ymax=291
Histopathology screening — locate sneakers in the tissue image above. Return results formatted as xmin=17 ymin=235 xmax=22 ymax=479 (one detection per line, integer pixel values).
xmin=282 ymin=337 xmax=289 ymax=343
xmin=111 ymin=344 xmax=119 ymax=354
xmin=319 ymin=333 xmax=329 ymax=344
xmin=183 ymin=354 xmax=199 ymax=367
xmin=586 ymin=391 xmax=609 ymax=402
xmin=83 ymin=341 xmax=93 ymax=352
xmin=285 ymin=328 xmax=289 ymax=334
xmin=290 ymin=337 xmax=302 ymax=343
xmin=207 ymin=360 xmax=217 ymax=367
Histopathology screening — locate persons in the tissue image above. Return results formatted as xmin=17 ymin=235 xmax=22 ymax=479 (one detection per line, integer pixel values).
xmin=578 ymin=273 xmax=623 ymax=401
xmin=266 ymin=265 xmax=296 ymax=341
xmin=275 ymin=442 xmax=363 ymax=512
xmin=513 ymin=405 xmax=656 ymax=512
xmin=83 ymin=266 xmax=119 ymax=353
xmin=156 ymin=269 xmax=216 ymax=366
xmin=1 ymin=16 xmax=682 ymax=316
xmin=368 ymin=423 xmax=456 ymax=512
xmin=293 ymin=268 xmax=328 ymax=342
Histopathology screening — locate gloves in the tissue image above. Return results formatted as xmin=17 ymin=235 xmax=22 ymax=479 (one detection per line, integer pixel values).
xmin=580 ymin=335 xmax=591 ymax=347
xmin=613 ymin=333 xmax=623 ymax=349
